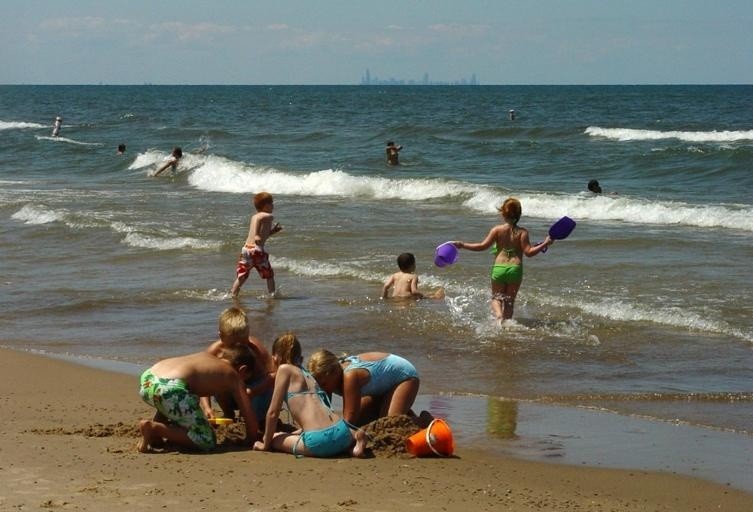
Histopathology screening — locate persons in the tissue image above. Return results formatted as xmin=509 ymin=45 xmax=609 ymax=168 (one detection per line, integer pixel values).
xmin=138 ymin=341 xmax=260 ymax=453
xmin=251 ymin=330 xmax=368 ymax=459
xmin=306 ymin=348 xmax=434 ymax=428
xmin=151 ymin=145 xmax=182 ymax=177
xmin=452 ymin=197 xmax=554 ymax=326
xmin=381 ymin=253 xmax=444 ymax=306
xmin=585 ymin=179 xmax=618 ymax=196
xmin=199 ymin=306 xmax=276 ymax=428
xmin=114 ymin=142 xmax=129 ymax=156
xmin=385 ymin=140 xmax=404 ymax=166
xmin=230 ymin=192 xmax=284 ymax=299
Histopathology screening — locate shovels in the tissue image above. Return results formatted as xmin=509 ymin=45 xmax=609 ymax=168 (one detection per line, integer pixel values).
xmin=535 ymin=216 xmax=576 ymax=253
